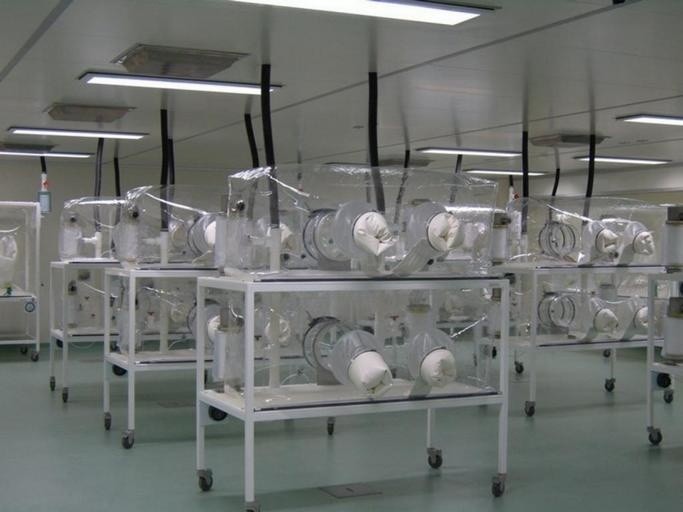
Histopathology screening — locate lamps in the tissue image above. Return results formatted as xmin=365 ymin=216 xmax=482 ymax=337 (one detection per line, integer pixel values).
xmin=42 ymin=101 xmax=137 ymax=123
xmin=615 ymin=113 xmax=682 ymax=127
xmin=415 ymin=147 xmax=523 ymax=159
xmin=461 ymin=169 xmax=548 ymax=177
xmin=242 ymin=1 xmax=503 ymax=27
xmin=573 ymin=154 xmax=672 ymax=167
xmin=78 ymin=70 xmax=286 ymax=97
xmin=6 ymin=126 xmax=150 ymax=141
xmin=108 ymin=42 xmax=251 ymax=80
xmin=0 ymin=147 xmax=95 ymax=160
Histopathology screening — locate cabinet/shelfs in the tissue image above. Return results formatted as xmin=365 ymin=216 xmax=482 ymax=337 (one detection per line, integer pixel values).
xmin=0 ymin=198 xmax=42 ymax=365
